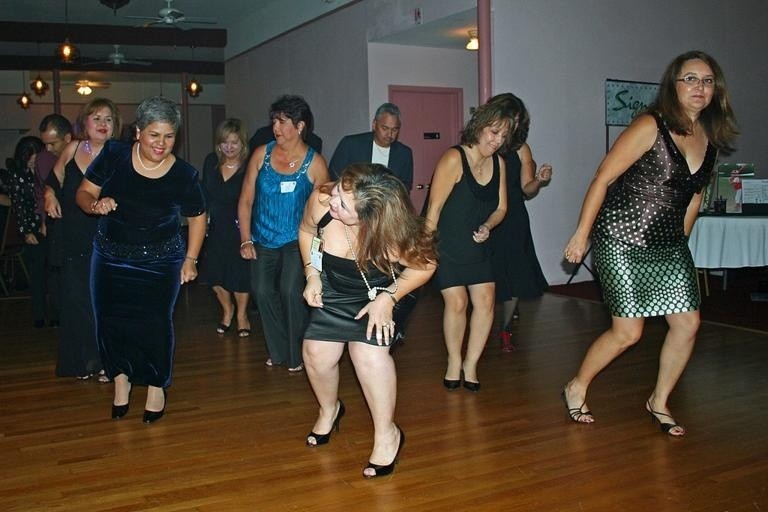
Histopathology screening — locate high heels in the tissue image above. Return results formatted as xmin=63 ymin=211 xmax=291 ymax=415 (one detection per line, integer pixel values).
xmin=306 ymin=399 xmax=346 ymax=446
xmin=364 ymin=422 xmax=405 ymax=479
xmin=501 ymin=331 xmax=514 ymax=353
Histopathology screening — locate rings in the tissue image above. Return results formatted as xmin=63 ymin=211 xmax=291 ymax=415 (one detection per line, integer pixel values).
xmin=382 ymin=323 xmax=390 ymax=328
xmin=47 ymin=212 xmax=51 ymax=216
xmin=389 ymin=320 xmax=397 ymax=328
xmin=101 ymin=202 xmax=109 ymax=210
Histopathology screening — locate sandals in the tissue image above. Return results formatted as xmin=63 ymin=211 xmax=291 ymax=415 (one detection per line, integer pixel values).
xmin=560 ymin=387 xmax=596 ymax=426
xmin=289 ymin=364 xmax=304 ymax=372
xmin=643 ymin=398 xmax=687 ymax=440
xmin=267 ymin=356 xmax=273 ymax=366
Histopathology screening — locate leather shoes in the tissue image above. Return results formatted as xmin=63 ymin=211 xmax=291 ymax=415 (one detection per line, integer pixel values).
xmin=461 ymin=368 xmax=480 ymax=393
xmin=110 ymin=380 xmax=133 ymax=420
xmin=443 ymin=367 xmax=461 ymax=392
xmin=143 ymin=388 xmax=167 ymax=422
xmin=512 ymin=308 xmax=518 ymax=319
xmin=33 ymin=318 xmax=42 ymax=326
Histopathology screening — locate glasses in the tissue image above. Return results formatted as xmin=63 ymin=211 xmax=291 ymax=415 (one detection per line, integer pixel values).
xmin=675 ymin=74 xmax=717 ymax=87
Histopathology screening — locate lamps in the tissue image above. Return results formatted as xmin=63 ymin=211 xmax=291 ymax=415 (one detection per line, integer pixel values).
xmin=465 ymin=28 xmax=480 ymax=51
xmin=16 ymin=70 xmax=34 ymax=110
xmin=185 ymin=46 xmax=203 ymax=98
xmin=56 ymin=0 xmax=79 ymax=67
xmin=30 ymin=43 xmax=49 ymax=99
xmin=74 ymin=80 xmax=91 ymax=95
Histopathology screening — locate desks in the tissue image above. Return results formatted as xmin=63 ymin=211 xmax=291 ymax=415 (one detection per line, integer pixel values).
xmin=688 ymin=216 xmax=767 ymax=296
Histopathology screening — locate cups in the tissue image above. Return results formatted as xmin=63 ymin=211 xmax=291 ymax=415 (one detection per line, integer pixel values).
xmin=713 ymin=199 xmax=726 ymax=217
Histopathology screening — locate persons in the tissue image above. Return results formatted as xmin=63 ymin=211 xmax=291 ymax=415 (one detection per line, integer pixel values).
xmin=481 ymin=94 xmax=554 ymax=353
xmin=40 ymin=96 xmax=120 ymax=385
xmin=425 ymin=104 xmax=510 ymax=392
xmin=562 ymin=51 xmax=742 ymax=438
xmin=202 ymin=119 xmax=252 ymax=338
xmin=237 ymin=94 xmax=329 ymax=373
xmin=33 ymin=113 xmax=74 ymax=238
xmin=74 ymin=95 xmax=207 ymax=423
xmin=297 ymin=160 xmax=440 ymax=482
xmin=328 ymin=102 xmax=413 ymax=191
xmin=9 ymin=135 xmax=61 ymax=328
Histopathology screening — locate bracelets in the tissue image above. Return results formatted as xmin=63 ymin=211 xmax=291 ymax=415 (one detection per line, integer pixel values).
xmin=238 ymin=240 xmax=254 ymax=247
xmin=84 ymin=198 xmax=104 ymax=216
xmin=306 ymin=272 xmax=320 ymax=280
xmin=385 ymin=291 xmax=399 ymax=305
xmin=302 ymin=271 xmax=325 ymax=280
xmin=183 ymin=255 xmax=199 ymax=264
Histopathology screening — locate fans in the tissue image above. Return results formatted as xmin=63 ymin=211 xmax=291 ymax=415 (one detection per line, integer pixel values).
xmin=81 ymin=45 xmax=152 ymax=68
xmin=123 ymin=0 xmax=218 ymax=30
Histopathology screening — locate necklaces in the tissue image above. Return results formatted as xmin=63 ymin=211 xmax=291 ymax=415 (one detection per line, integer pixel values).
xmin=289 ymin=160 xmax=297 ymax=168
xmin=83 ymin=139 xmax=101 ymax=155
xmin=136 ymin=143 xmax=167 ymax=171
xmin=226 ymin=163 xmax=237 ymax=169
xmin=344 ymin=222 xmax=398 ymax=302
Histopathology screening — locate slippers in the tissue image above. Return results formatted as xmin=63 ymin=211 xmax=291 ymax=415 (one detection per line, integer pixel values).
xmin=218 ymin=322 xmax=227 ymax=335
xmin=236 ymin=326 xmax=252 ymax=338
